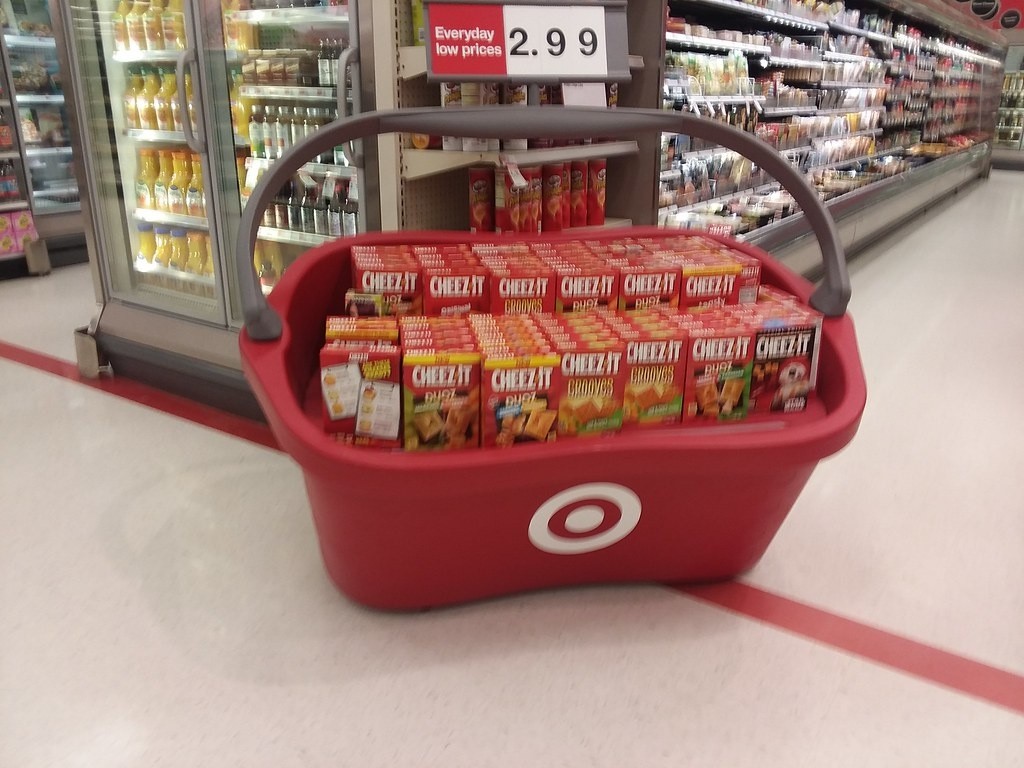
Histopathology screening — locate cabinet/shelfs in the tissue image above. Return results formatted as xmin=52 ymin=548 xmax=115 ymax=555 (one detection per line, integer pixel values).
xmin=991 ymin=70 xmax=1024 ymax=171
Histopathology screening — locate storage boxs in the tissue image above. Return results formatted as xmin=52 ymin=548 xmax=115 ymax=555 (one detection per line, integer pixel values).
xmin=300 ymin=234 xmax=825 ymax=454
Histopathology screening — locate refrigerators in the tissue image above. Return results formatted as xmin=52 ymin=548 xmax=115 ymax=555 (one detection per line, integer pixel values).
xmin=46 ymin=1 xmax=380 ymax=425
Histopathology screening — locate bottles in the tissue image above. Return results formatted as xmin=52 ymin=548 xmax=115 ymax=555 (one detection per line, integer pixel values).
xmin=0 ymin=111 xmax=21 ymax=204
xmin=104 ymin=0 xmax=359 ymax=300
